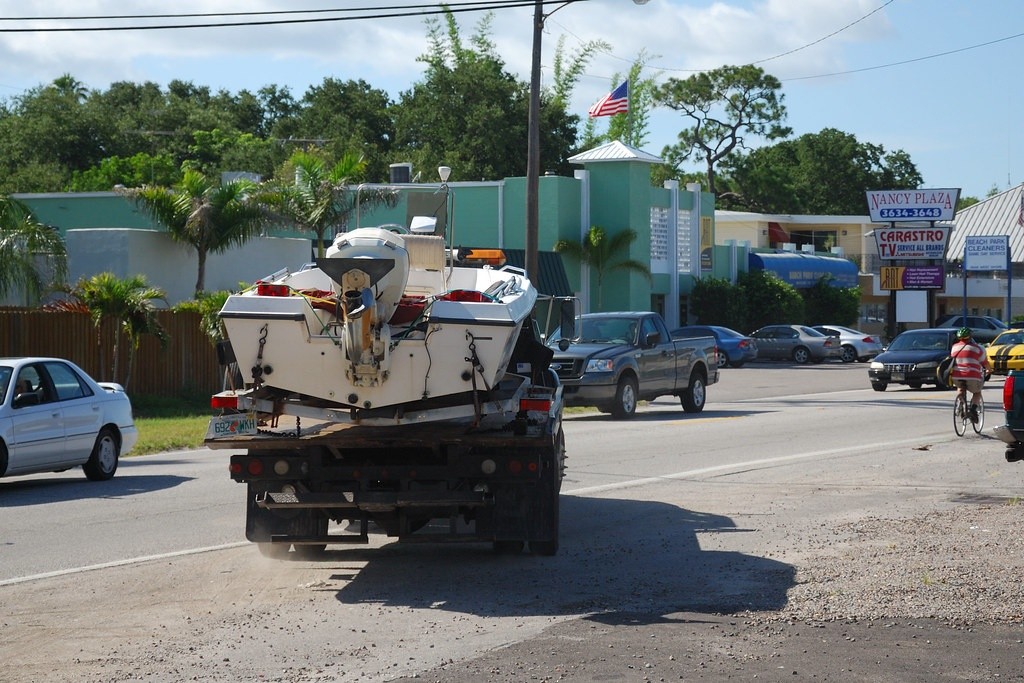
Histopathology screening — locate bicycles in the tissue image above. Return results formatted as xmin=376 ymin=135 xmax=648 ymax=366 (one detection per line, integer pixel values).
xmin=953 ymin=370 xmax=989 ymax=436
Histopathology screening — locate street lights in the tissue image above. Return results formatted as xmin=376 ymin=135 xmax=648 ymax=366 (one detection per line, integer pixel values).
xmin=523 ymin=0 xmax=650 ymax=293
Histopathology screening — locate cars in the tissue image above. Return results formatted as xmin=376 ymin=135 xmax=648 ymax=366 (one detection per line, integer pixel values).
xmin=939 ymin=314 xmax=1013 ymax=343
xmin=982 ymin=330 xmax=1024 ymax=381
xmin=868 ymin=329 xmax=959 ymax=391
xmin=748 ymin=324 xmax=844 ymax=364
xmin=669 ymin=324 xmax=758 ymax=369
xmin=792 ymin=324 xmax=883 ymax=363
xmin=0 ymin=354 xmax=135 ymax=483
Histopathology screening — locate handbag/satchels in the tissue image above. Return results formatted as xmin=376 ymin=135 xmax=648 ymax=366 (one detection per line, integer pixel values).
xmin=946 ymin=358 xmax=956 ymax=380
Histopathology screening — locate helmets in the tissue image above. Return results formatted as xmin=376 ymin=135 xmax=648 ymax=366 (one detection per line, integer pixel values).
xmin=957 ymin=328 xmax=973 ymax=339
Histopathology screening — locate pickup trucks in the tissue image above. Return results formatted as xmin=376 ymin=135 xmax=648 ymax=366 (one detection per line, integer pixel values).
xmin=993 ymin=369 xmax=1024 ymax=448
xmin=543 ymin=310 xmax=720 ymax=419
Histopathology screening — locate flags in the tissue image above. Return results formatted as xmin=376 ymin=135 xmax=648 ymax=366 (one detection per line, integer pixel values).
xmin=588 ymin=80 xmax=628 ymax=117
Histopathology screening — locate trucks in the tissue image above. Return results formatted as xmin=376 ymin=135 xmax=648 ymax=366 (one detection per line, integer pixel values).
xmin=202 ymin=250 xmax=584 ymax=559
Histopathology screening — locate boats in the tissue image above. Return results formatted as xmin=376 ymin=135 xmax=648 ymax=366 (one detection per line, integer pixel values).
xmin=214 ymin=216 xmax=539 ymax=414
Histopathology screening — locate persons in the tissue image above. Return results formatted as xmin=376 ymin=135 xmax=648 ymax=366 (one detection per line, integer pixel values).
xmin=627 ymin=322 xmax=647 ymax=346
xmin=949 ymin=326 xmax=994 ymax=424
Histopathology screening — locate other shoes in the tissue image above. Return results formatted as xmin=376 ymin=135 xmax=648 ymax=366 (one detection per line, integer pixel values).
xmin=970 ymin=408 xmax=979 ymax=423
xmin=955 ymin=401 xmax=963 ymax=416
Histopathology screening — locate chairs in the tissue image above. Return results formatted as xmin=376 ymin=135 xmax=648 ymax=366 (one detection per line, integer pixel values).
xmin=396 ymin=234 xmax=448 ymax=293
xmin=16 ymin=379 xmax=33 ymax=392
xmin=580 ymin=325 xmax=601 ymax=340
